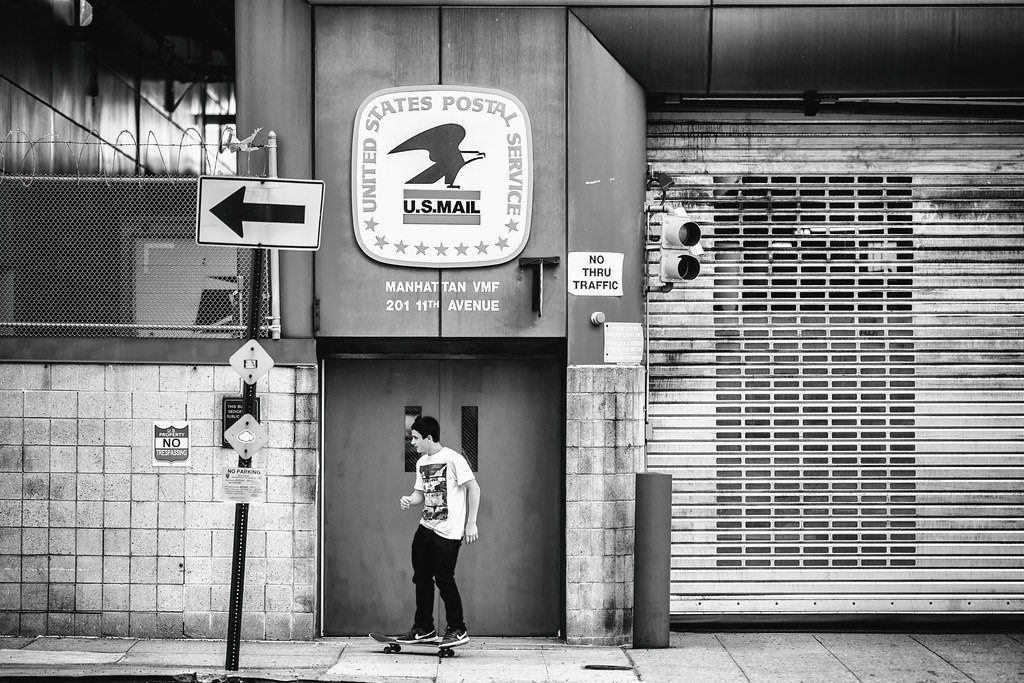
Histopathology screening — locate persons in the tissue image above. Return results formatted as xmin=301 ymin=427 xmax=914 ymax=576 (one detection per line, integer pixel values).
xmin=395 ymin=416 xmax=481 ymax=648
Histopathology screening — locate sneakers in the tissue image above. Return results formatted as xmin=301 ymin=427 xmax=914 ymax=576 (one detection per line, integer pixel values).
xmin=438 ymin=624 xmax=470 ymax=646
xmin=396 ymin=624 xmax=439 ymax=644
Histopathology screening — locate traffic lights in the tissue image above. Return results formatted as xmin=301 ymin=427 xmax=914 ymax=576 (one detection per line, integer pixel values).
xmin=659 ymin=215 xmax=702 ymax=283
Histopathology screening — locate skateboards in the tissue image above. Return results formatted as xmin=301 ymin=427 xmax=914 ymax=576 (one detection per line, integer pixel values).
xmin=369 ymin=633 xmax=467 ymax=659
